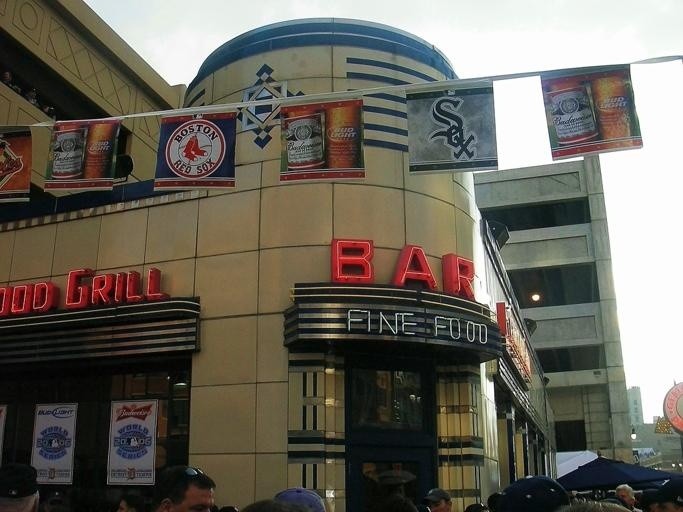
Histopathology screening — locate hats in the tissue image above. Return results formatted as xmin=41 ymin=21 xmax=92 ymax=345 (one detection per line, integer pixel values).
xmin=421 ymin=486 xmax=451 ymax=503
xmin=0 ymin=461 xmax=39 ymax=499
xmin=502 ymin=473 xmax=571 ymax=512
xmin=273 ymin=486 xmax=327 ymax=512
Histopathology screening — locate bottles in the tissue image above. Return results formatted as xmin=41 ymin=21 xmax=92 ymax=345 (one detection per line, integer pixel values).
xmin=545 ymin=78 xmax=598 ymax=149
xmin=282 ymin=110 xmax=325 ymax=170
xmin=590 ymin=73 xmax=631 ymax=148
xmin=52 ymin=124 xmax=84 ymax=180
xmin=82 ymin=121 xmax=117 ymax=178
xmin=324 ymin=107 xmax=361 ymax=168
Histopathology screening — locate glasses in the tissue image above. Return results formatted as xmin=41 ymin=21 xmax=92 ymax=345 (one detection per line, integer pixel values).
xmin=169 ymin=466 xmax=204 ymax=500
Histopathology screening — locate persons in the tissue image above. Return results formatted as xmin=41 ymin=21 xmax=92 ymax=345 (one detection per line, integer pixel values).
xmin=3 ymin=463 xmax=683 ymax=511
